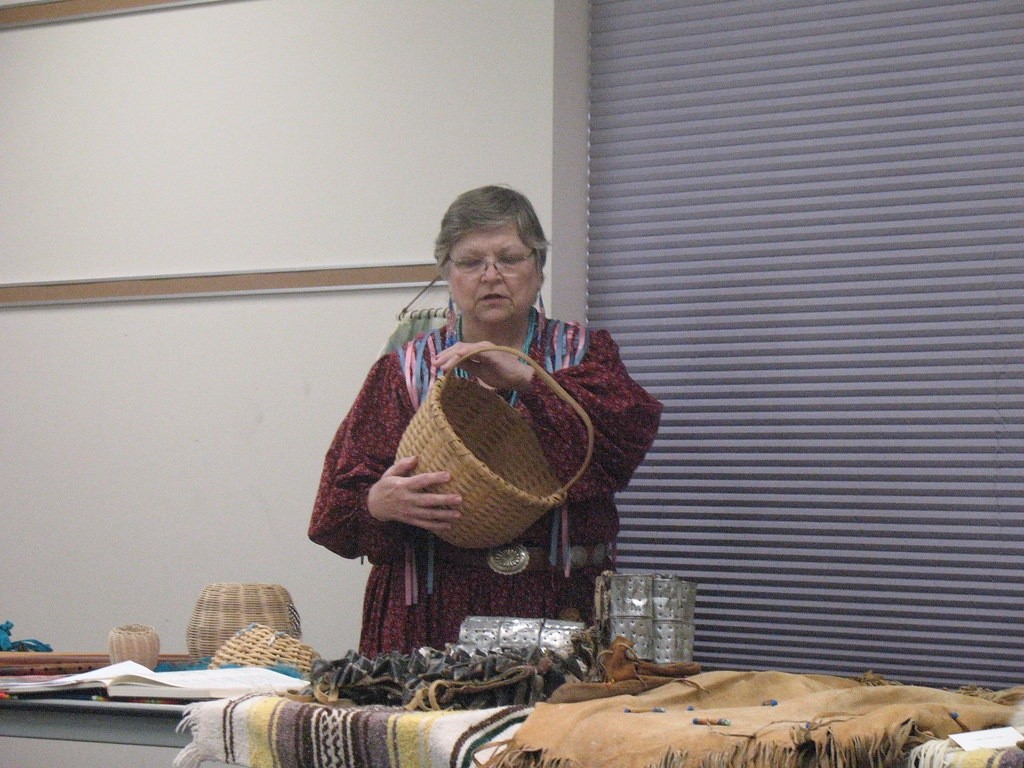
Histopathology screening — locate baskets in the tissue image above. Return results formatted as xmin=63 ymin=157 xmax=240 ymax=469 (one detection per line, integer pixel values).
xmin=394 ymin=344 xmax=595 ymax=551
xmin=207 ymin=623 xmax=321 ymax=680
xmin=185 ymin=583 xmax=301 ymax=660
xmin=108 ymin=623 xmax=161 ymax=669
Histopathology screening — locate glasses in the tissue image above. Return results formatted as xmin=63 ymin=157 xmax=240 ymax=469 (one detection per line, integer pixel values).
xmin=447 ymin=246 xmax=535 ymax=272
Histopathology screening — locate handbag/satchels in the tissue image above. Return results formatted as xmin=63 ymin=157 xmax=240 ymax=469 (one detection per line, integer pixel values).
xmin=378 ymin=275 xmax=458 ymax=355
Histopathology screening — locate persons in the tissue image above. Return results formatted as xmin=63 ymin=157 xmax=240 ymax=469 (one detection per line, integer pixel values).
xmin=309 ymin=187 xmax=663 ymax=658
